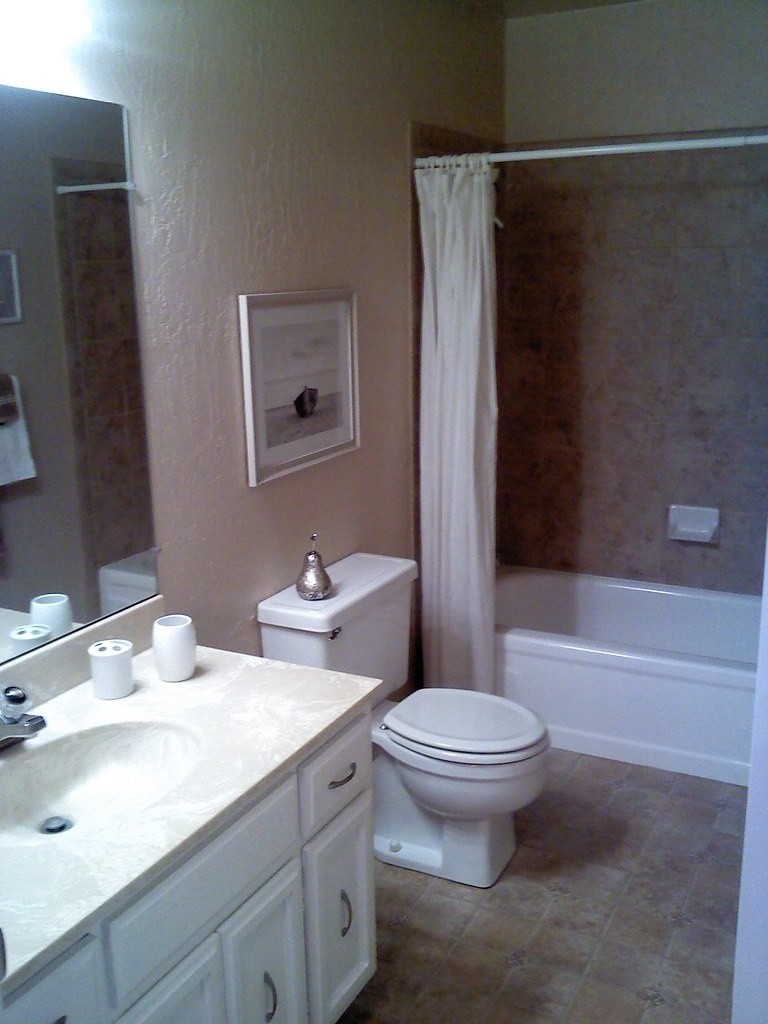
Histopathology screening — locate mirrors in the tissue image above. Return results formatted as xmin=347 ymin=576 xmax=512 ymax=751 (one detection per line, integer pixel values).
xmin=0 ymin=83 xmax=160 ymax=665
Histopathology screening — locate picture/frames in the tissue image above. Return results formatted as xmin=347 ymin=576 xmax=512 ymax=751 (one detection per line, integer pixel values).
xmin=237 ymin=288 xmax=363 ymax=489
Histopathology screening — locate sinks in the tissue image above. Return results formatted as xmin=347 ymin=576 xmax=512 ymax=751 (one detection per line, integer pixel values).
xmin=0 ymin=709 xmax=211 ymax=846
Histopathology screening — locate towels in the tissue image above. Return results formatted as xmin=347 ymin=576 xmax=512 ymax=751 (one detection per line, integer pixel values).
xmin=0 ymin=372 xmax=39 ymax=487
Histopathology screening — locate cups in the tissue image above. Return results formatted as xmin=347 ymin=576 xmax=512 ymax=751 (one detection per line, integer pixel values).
xmin=29 ymin=594 xmax=72 ymax=638
xmin=152 ymin=614 xmax=198 ymax=682
xmin=11 ymin=623 xmax=52 ymax=658
xmin=89 ymin=638 xmax=135 ymax=700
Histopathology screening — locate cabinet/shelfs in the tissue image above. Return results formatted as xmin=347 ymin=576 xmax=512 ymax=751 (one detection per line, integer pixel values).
xmin=0 ymin=706 xmax=378 ymax=1024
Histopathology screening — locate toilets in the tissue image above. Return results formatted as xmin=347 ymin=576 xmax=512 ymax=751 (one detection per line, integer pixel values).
xmin=252 ymin=550 xmax=553 ymax=888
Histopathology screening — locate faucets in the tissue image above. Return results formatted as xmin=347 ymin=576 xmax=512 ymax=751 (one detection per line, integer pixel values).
xmin=1 ymin=683 xmax=49 ymax=751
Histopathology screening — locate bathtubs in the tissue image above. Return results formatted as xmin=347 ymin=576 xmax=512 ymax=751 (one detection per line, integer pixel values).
xmin=492 ymin=562 xmax=762 ymax=787
